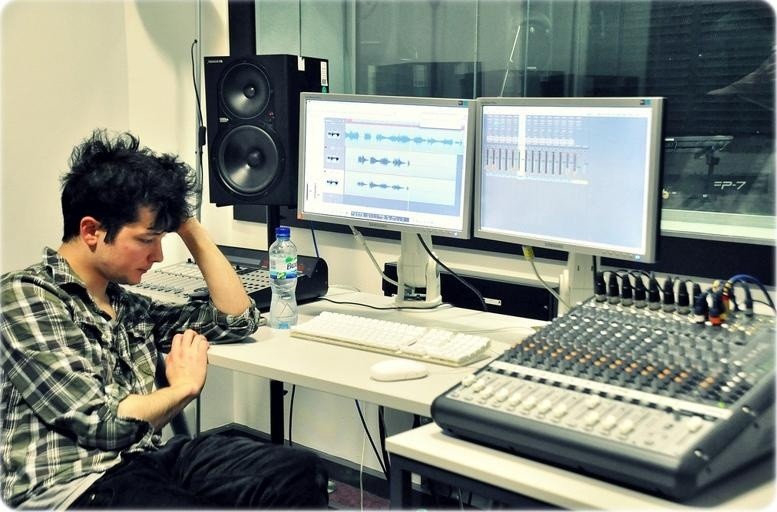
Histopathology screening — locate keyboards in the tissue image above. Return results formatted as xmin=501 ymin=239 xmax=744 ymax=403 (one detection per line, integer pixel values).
xmin=291 ymin=311 xmax=492 ymax=368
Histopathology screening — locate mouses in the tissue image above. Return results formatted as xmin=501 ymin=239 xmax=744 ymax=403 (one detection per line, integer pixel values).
xmin=371 ymin=360 xmax=427 ymax=381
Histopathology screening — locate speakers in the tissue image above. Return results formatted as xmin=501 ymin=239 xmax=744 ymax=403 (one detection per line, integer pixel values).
xmin=204 ymin=54 xmax=329 ymax=208
xmin=376 ymin=61 xmax=483 ymax=98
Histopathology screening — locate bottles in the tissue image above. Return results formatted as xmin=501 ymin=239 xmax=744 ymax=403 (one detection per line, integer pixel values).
xmin=268 ymin=226 xmax=298 ymax=329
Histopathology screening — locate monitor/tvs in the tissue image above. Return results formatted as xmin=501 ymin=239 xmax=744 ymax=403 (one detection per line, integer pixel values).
xmin=476 ymin=96 xmax=664 ymax=316
xmin=296 ymin=91 xmax=476 ymax=312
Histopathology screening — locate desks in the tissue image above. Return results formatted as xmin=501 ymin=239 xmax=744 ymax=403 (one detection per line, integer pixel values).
xmin=186 ymin=285 xmax=776 ymax=511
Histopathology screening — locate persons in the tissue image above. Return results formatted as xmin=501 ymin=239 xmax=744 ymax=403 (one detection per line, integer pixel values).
xmin=0 ymin=130 xmax=328 ymax=510
